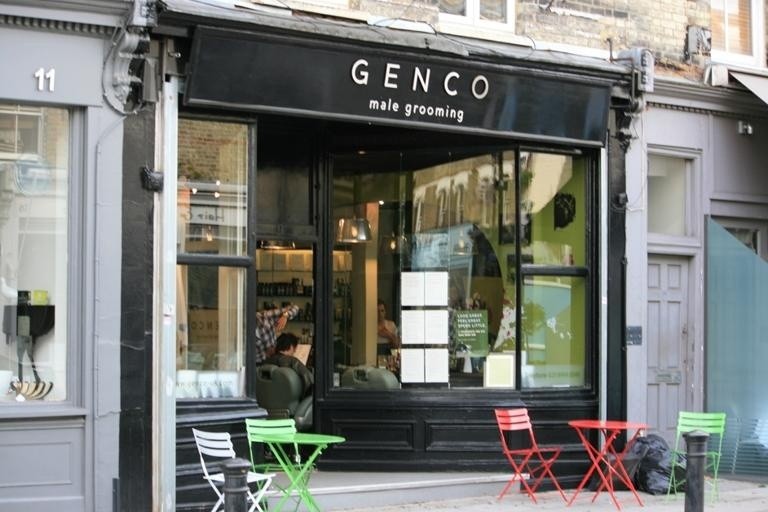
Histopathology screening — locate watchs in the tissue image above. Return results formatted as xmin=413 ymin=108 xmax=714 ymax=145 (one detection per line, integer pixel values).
xmin=283 ymin=311 xmax=289 ymax=318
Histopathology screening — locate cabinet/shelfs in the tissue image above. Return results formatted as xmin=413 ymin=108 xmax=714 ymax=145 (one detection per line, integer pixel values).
xmin=251 ymin=234 xmax=356 ymax=375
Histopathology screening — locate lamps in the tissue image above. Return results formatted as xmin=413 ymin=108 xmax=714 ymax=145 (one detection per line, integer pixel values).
xmin=333 ymin=168 xmax=374 ymax=245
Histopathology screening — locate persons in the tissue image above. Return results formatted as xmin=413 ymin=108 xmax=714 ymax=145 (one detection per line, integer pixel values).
xmin=263 ymin=333 xmax=314 ymax=404
xmin=254 ymin=304 xmax=300 ymax=366
xmin=378 ymin=298 xmax=400 ymax=374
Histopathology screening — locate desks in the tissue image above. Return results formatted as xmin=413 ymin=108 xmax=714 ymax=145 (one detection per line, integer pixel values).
xmin=565 ymin=417 xmax=656 ymax=512
xmin=247 ymin=430 xmax=347 ymax=512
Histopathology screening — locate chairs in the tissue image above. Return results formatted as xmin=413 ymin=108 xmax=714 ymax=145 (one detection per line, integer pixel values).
xmin=190 ymin=424 xmax=278 ymax=512
xmin=253 ymin=361 xmax=313 ymax=463
xmin=490 ymin=405 xmax=569 ymax=508
xmin=663 ymin=409 xmax=727 ymax=507
xmin=242 ymin=416 xmax=318 ymax=512
xmin=338 ymin=361 xmax=406 ymax=393
